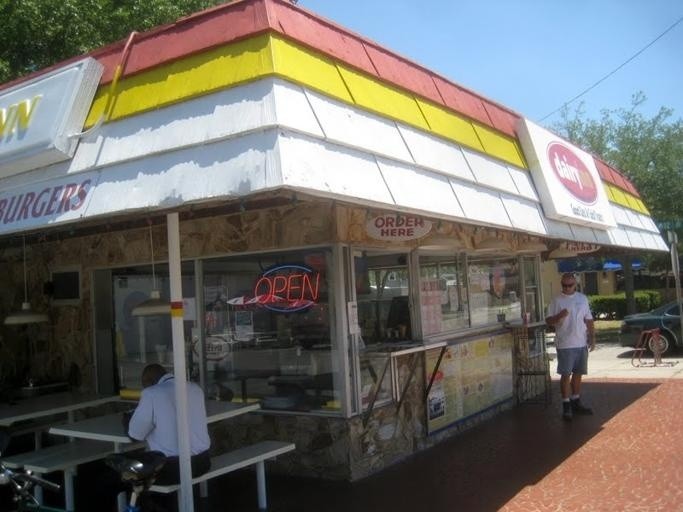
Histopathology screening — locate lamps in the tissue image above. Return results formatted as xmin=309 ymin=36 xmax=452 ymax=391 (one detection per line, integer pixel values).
xmin=4 ymin=234 xmax=49 ymax=326
xmin=127 ymin=225 xmax=171 ymax=317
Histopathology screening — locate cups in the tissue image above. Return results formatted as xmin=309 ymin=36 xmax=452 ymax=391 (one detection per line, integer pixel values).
xmin=384 ymin=324 xmax=408 ymax=341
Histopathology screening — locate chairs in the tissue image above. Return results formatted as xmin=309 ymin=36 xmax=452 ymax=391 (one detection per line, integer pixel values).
xmin=509 ymin=324 xmax=553 ymax=409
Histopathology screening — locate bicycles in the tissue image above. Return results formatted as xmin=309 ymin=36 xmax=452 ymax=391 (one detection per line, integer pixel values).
xmin=0 ymin=442 xmax=168 ymax=512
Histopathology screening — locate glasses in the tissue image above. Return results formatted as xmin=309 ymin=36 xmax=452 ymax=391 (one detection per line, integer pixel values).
xmin=562 ymin=283 xmax=575 ymax=288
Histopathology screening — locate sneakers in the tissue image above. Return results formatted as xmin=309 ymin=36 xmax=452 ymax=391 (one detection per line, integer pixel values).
xmin=570 ymin=398 xmax=591 ymax=416
xmin=563 ymin=401 xmax=571 ymax=418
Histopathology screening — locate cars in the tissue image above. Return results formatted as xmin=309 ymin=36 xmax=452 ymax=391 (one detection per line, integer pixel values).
xmin=618 ymin=297 xmax=682 ymax=359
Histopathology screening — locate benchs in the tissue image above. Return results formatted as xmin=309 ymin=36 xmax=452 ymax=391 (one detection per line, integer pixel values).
xmin=3 ymin=443 xmax=69 ymax=474
xmin=24 ymin=443 xmax=146 ymax=511
xmin=150 ymin=440 xmax=295 ymax=511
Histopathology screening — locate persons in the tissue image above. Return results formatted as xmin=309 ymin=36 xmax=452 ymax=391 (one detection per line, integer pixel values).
xmin=545 ymin=272 xmax=595 ymax=423
xmin=117 ymin=363 xmax=211 ymax=511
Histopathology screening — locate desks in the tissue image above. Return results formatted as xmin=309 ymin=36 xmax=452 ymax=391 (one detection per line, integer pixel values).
xmin=48 ymin=400 xmax=263 ymax=512
xmin=0 ymin=394 xmax=121 ymax=504
